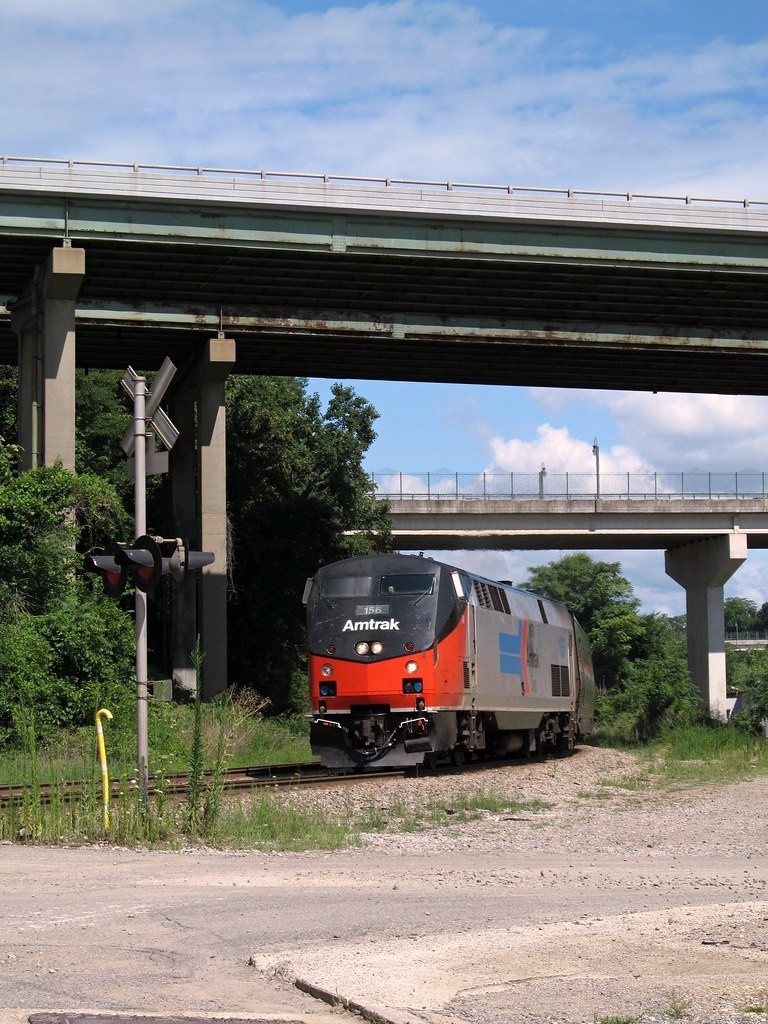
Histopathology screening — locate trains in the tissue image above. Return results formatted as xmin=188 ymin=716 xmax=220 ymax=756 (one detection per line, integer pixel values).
xmin=302 ymin=554 xmax=596 ymax=777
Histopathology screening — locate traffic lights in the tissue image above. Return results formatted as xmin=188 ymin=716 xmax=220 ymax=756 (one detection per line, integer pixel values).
xmin=170 ymin=538 xmax=216 ymax=585
xmin=84 ymin=535 xmax=163 ymax=596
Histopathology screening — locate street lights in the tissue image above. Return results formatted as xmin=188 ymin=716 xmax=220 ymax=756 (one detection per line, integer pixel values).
xmin=592 ymin=437 xmax=600 ymax=498
